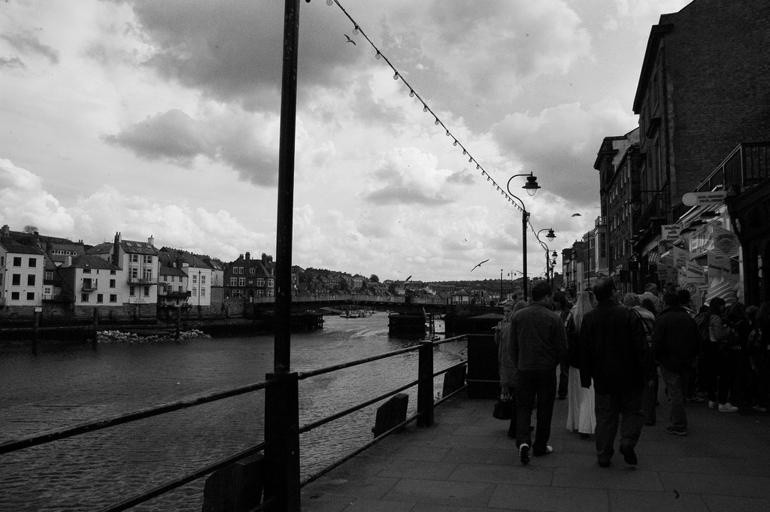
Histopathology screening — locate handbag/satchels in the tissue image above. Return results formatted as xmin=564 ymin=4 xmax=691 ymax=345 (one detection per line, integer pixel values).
xmin=493 ymin=399 xmax=510 ymax=420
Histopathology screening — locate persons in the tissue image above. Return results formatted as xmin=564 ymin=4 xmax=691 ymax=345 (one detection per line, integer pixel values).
xmin=508 ymin=282 xmax=568 ymax=465
xmin=579 ymin=278 xmax=651 ymax=467
xmin=498 ymin=283 xmax=770 ymax=437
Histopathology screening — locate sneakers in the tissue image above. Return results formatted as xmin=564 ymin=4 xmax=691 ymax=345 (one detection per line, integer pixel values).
xmin=519 ymin=443 xmax=529 ymax=464
xmin=666 ymin=426 xmax=687 ymax=436
xmin=708 ymin=401 xmax=767 ymax=413
xmin=509 ymin=426 xmax=535 ymax=438
xmin=686 ymin=395 xmax=705 ymax=403
xmin=535 ymin=446 xmax=553 ymax=456
xmin=619 ymin=444 xmax=638 ymax=465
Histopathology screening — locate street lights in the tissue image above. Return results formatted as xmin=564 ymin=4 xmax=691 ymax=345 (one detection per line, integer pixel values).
xmin=500 ymin=171 xmax=557 ymax=303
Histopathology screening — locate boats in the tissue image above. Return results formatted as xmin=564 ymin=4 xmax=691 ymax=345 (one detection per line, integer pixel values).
xmin=339 ymin=308 xmax=367 ymax=319
